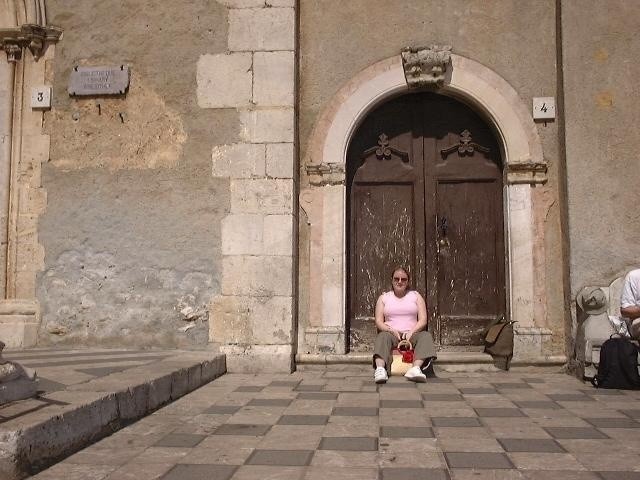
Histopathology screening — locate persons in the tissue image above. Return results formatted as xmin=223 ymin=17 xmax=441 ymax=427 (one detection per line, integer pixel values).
xmin=372 ymin=267 xmax=437 ymax=384
xmin=620 ymin=269 xmax=640 ymax=339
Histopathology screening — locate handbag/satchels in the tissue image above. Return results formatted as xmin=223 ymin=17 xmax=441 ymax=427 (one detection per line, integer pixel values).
xmin=480 ymin=313 xmax=513 ymax=357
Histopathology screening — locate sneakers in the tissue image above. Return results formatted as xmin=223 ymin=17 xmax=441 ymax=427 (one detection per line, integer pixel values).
xmin=374 ymin=367 xmax=388 ymax=383
xmin=404 ymin=366 xmax=427 ymax=382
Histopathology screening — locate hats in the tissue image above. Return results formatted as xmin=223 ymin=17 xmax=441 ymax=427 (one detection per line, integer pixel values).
xmin=577 ymin=286 xmax=608 ymax=314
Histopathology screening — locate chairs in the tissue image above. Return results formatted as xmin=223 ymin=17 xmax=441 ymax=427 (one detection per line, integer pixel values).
xmin=572 ymin=266 xmax=640 ymax=384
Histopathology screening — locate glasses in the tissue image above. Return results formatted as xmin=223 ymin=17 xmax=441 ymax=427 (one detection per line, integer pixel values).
xmin=393 ymin=277 xmax=407 ymax=283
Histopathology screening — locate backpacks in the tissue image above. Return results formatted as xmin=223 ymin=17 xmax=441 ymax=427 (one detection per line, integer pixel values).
xmin=591 ymin=333 xmax=640 ymax=390
